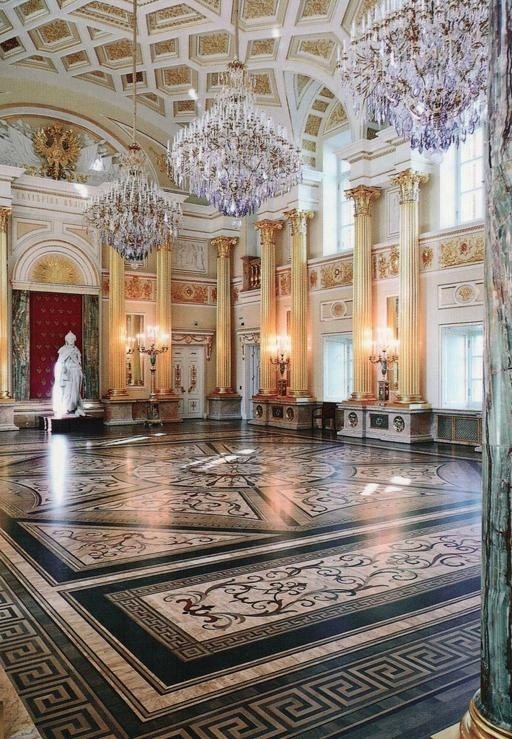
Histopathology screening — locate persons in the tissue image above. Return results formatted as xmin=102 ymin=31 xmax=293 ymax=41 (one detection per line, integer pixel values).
xmin=52 ymin=331 xmax=82 ymax=416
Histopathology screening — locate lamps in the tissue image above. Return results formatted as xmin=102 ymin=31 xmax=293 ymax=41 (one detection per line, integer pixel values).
xmin=83 ymin=2 xmax=185 ymax=268
xmin=333 ymin=1 xmax=491 ymax=157
xmin=166 ymin=1 xmax=304 ymax=219
